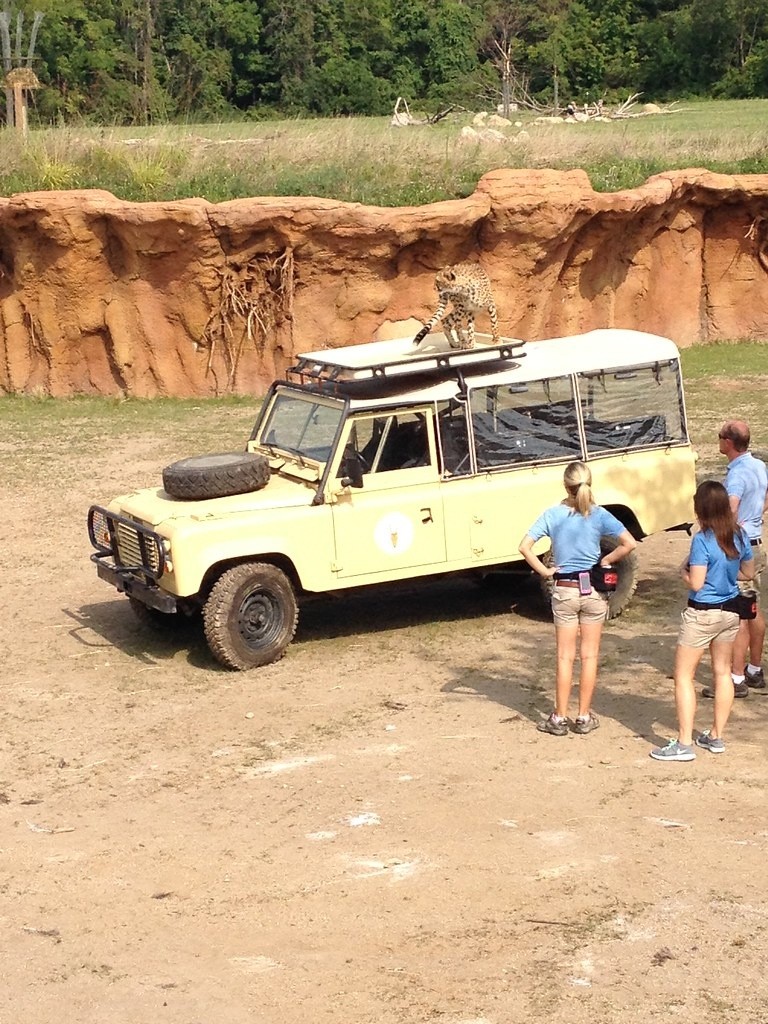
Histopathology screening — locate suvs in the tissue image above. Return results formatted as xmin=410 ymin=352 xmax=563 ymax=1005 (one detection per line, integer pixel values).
xmin=86 ymin=327 xmax=698 ymax=673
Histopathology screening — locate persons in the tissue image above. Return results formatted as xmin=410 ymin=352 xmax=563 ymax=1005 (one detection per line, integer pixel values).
xmin=702 ymin=418 xmax=768 ymax=700
xmin=356 ymin=417 xmax=425 ymax=472
xmin=518 ymin=460 xmax=639 ymax=735
xmin=650 ymin=478 xmax=755 ymax=762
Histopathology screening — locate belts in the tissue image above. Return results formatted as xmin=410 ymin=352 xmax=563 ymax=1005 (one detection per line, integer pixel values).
xmin=688 ymin=599 xmax=734 ymax=609
xmin=751 ymin=539 xmax=762 ymax=546
xmin=556 ymin=581 xmax=580 ymax=587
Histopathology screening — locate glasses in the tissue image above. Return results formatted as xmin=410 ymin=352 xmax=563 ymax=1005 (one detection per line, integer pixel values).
xmin=718 ymin=433 xmax=727 ymax=440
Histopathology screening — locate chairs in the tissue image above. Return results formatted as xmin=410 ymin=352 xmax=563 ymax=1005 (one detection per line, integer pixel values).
xmin=400 ymin=420 xmax=430 ymax=469
xmin=360 ymin=416 xmax=398 ymax=472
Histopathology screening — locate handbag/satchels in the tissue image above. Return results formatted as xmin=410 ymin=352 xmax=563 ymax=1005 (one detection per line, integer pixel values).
xmin=592 ymin=564 xmax=618 ymax=592
xmin=738 ymin=591 xmax=756 ymax=619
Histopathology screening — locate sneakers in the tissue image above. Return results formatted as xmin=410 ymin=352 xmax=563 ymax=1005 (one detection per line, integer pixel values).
xmin=575 ymin=711 xmax=599 ymax=734
xmin=744 ymin=664 xmax=766 ymax=688
xmin=695 ymin=729 xmax=726 ymax=753
xmin=536 ymin=711 xmax=569 ymax=736
xmin=649 ymin=738 xmax=697 ymax=761
xmin=702 ymin=679 xmax=749 ymax=698
